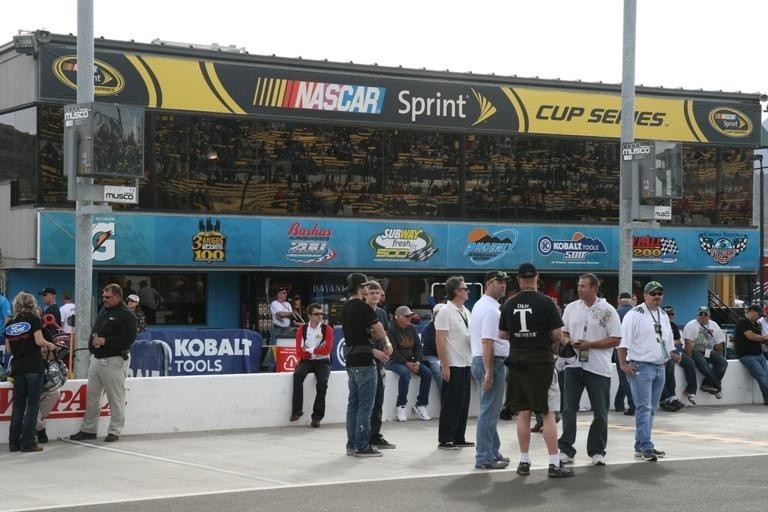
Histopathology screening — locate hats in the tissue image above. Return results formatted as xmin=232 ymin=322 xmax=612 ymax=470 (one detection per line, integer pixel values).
xmin=126 ymin=294 xmax=140 ymax=303
xmin=697 ymin=306 xmax=711 ymax=316
xmin=643 ymin=281 xmax=664 ymax=294
xmin=343 ymin=273 xmax=373 ymax=293
xmin=747 ymin=305 xmax=766 ymax=317
xmin=37 ymin=287 xmax=57 ymax=295
xmin=519 ymin=263 xmax=537 ymax=279
xmin=555 ymin=342 xmax=577 ymax=370
xmin=484 ymin=271 xmax=513 ymax=285
xmin=620 ymin=293 xmax=631 ymax=298
xmin=395 ymin=306 xmax=416 ymax=318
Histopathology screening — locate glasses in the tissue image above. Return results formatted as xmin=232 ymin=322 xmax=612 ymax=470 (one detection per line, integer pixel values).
xmin=699 ymin=313 xmax=708 ymax=316
xmin=486 ymin=271 xmax=507 ymax=281
xmin=127 ymin=299 xmax=134 ymax=302
xmin=458 ymin=288 xmax=470 ymax=291
xmin=101 ymin=295 xmax=116 ymax=299
xmin=311 ymin=313 xmax=323 ymax=315
xmin=649 ymin=292 xmax=663 ymax=296
xmin=279 ymin=287 xmax=286 ymax=290
xmin=667 ymin=312 xmax=674 ymax=316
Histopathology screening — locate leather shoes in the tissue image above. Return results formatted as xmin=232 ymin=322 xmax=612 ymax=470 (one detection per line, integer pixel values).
xmin=105 ymin=434 xmax=119 ymax=443
xmin=311 ymin=419 xmax=321 ymax=428
xmin=70 ymin=431 xmax=97 ymax=441
xmin=21 ymin=446 xmax=43 ymax=452
xmin=624 ymin=407 xmax=634 ymax=416
xmin=290 ymin=411 xmax=303 ymax=422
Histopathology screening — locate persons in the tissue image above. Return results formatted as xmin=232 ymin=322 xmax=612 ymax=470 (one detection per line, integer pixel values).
xmin=343 ymin=263 xmax=768 ymax=478
xmin=291 ymin=294 xmax=305 ymax=326
xmin=0 ymin=271 xmax=75 ymax=453
xmin=290 ymin=302 xmax=334 ymax=428
xmin=127 ymin=273 xmax=205 ymax=330
xmin=268 ymin=288 xmax=292 ymax=373
xmin=70 ymin=283 xmax=137 ymax=443
xmin=127 ymin=294 xmax=146 ymax=334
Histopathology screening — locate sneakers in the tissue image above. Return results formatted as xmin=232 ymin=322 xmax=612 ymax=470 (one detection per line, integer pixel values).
xmin=36 ymin=428 xmax=48 ymax=444
xmin=476 ymin=449 xmax=666 ymax=478
xmin=438 ymin=442 xmax=459 ymax=450
xmin=454 ymin=442 xmax=476 ymax=448
xmin=411 ymin=404 xmax=432 ymax=422
xmin=540 ymin=411 xmax=561 ymax=431
xmin=531 ymin=422 xmax=543 ymax=432
xmin=658 ymin=385 xmax=723 ymax=412
xmin=396 ymin=405 xmax=407 ymax=422
xmin=346 ymin=435 xmax=396 ymax=458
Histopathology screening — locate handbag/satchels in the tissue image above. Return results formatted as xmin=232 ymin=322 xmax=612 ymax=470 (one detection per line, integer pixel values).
xmin=40 ymin=348 xmax=69 ymax=393
